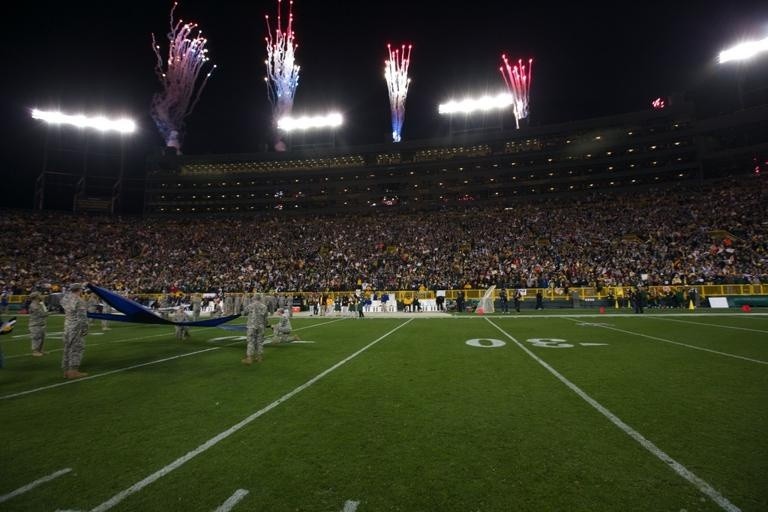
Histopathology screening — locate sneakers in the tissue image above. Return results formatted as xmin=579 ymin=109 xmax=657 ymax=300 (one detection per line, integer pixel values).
xmin=295 ymin=335 xmax=300 ymax=341
xmin=242 ymin=358 xmax=252 ymax=364
xmin=63 ymin=371 xmax=81 ymax=379
xmin=33 ymin=350 xmax=43 ymax=356
xmin=102 ymin=327 xmax=111 ymax=331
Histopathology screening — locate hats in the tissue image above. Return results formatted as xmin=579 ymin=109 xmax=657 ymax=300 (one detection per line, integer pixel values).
xmin=70 ymin=283 xmax=84 ymax=290
xmin=30 ymin=292 xmax=40 ymax=297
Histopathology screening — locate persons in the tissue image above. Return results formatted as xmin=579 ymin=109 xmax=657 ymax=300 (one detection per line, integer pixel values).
xmin=0 ymin=320 xmax=16 ymax=335
xmin=172 ymin=307 xmax=190 ymax=344
xmin=1 ymin=160 xmax=767 ymax=314
xmin=58 ymin=281 xmax=90 ymax=380
xmin=270 ymin=308 xmax=301 ymax=345
xmin=239 ymin=294 xmax=269 ymax=365
xmin=26 ymin=288 xmax=51 ymax=358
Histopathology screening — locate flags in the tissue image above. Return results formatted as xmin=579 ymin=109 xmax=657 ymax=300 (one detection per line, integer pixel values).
xmin=83 ymin=283 xmax=241 ymax=328
